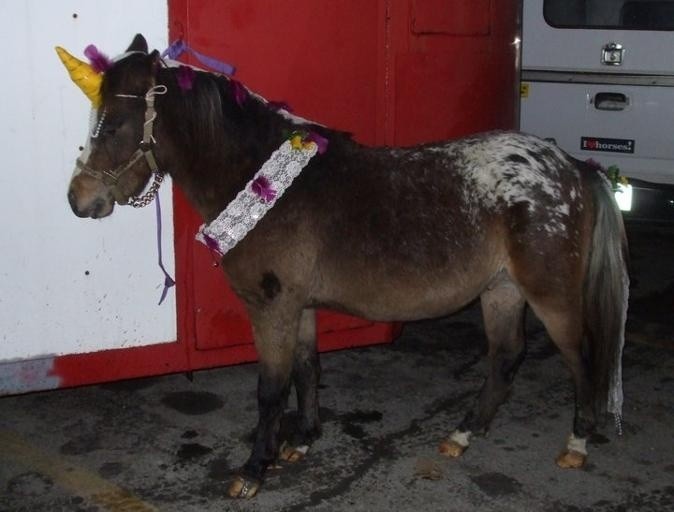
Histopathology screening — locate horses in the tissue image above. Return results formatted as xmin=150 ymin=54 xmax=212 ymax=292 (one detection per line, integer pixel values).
xmin=67 ymin=33 xmax=632 ymax=500
